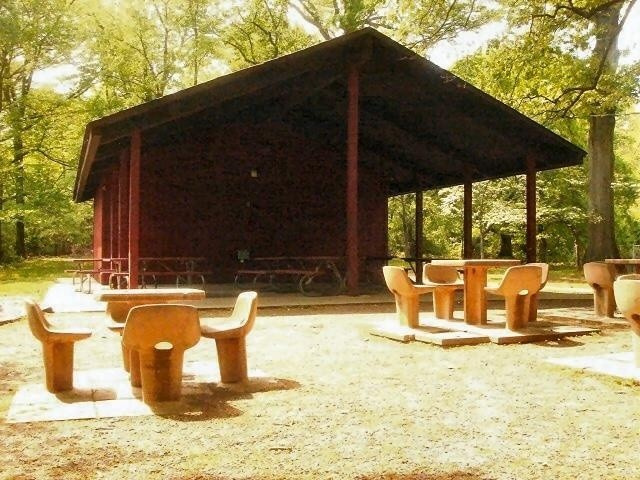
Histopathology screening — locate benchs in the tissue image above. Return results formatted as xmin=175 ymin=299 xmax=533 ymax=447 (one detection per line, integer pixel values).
xmin=67 ymin=257 xmax=214 ymax=294
xmin=234 ymin=256 xmax=432 ymax=296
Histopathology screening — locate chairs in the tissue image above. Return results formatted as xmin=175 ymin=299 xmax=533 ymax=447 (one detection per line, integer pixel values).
xmin=382 ymin=252 xmax=640 ymax=345
xmin=20 ymin=282 xmax=263 ymax=403
